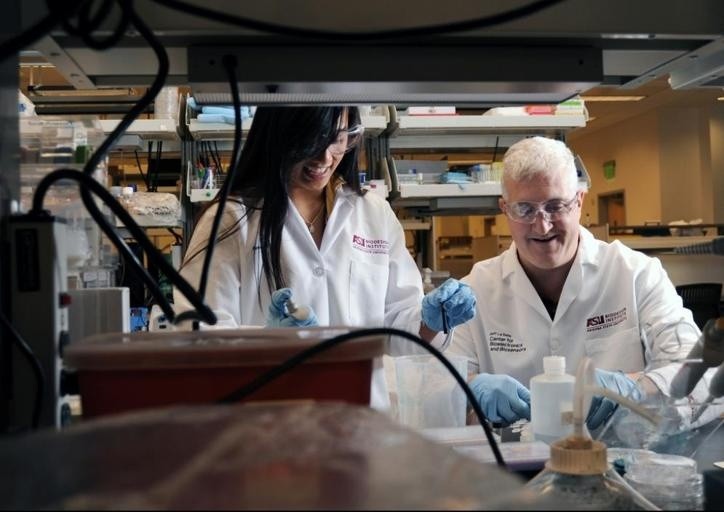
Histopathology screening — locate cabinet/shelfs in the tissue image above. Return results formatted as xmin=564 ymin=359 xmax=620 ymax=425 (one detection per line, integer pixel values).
xmin=17 ymin=97 xmax=587 ymax=331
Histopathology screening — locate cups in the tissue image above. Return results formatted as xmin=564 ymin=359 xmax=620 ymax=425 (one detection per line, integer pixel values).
xmin=394 ymin=351 xmax=469 ymax=432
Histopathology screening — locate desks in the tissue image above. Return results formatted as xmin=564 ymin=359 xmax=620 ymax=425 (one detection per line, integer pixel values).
xmin=0 ymin=399 xmax=591 ymax=511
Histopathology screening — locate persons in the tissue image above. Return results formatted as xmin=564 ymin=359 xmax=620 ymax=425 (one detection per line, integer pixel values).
xmin=421 ymin=135 xmax=720 ymax=431
xmin=171 ymin=103 xmax=479 ymax=424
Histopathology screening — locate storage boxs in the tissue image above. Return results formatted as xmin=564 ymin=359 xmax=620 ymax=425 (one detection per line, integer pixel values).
xmin=65 ymin=327 xmax=384 ymax=420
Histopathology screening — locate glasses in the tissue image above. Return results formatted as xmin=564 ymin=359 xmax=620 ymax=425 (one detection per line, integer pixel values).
xmin=322 ymin=123 xmax=366 ymax=156
xmin=499 ymin=192 xmax=582 ymax=226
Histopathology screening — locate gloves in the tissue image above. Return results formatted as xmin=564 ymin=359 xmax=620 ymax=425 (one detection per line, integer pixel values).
xmin=419 ymin=275 xmax=479 ymax=334
xmin=584 ymin=366 xmax=644 ymax=431
xmin=465 ymin=372 xmax=534 ymax=428
xmin=262 ymin=287 xmax=321 ymax=330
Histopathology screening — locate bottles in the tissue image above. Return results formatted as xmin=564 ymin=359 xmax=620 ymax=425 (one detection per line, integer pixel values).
xmin=528 ymin=355 xmax=577 ymax=445
